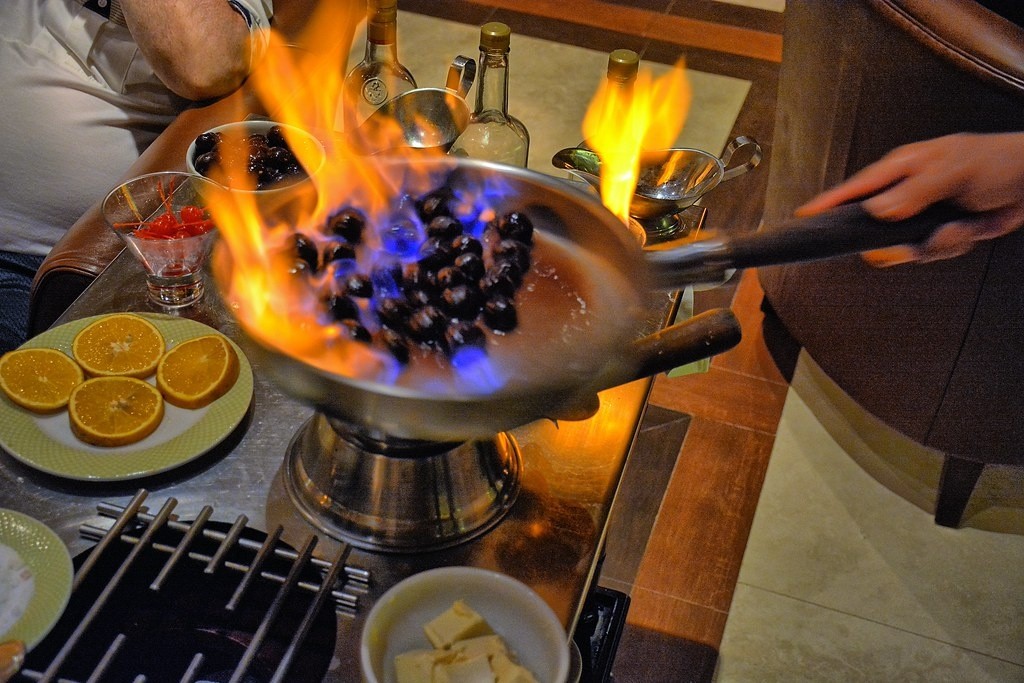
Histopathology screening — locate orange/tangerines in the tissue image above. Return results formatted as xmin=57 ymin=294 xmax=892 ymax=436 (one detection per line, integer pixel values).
xmin=72 ymin=314 xmax=167 ymax=380
xmin=154 ymin=335 xmax=239 ymax=410
xmin=67 ymin=374 xmax=164 ymax=446
xmin=0 ymin=348 xmax=84 ymax=415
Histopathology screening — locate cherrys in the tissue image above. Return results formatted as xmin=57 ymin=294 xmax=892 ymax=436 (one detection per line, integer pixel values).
xmin=111 ymin=179 xmax=215 ymax=275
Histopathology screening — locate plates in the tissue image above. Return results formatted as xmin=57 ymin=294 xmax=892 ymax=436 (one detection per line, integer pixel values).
xmin=0 ymin=311 xmax=254 ymax=482
xmin=0 ymin=507 xmax=74 ymax=656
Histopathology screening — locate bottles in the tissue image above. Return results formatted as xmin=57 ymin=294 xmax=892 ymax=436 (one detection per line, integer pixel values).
xmin=605 ymin=48 xmax=639 ymax=107
xmin=342 ymin=0 xmax=418 ymax=133
xmin=453 ymin=20 xmax=529 ymax=171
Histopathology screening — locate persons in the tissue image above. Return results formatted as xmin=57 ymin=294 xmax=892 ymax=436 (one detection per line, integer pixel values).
xmin=796 ymin=131 xmax=1023 ymax=269
xmin=1 ymin=0 xmax=273 ymax=357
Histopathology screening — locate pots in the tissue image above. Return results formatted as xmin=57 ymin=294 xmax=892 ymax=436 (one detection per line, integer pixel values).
xmin=207 ymin=155 xmax=982 ymax=444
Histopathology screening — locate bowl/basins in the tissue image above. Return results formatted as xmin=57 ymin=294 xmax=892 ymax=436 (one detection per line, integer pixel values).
xmin=359 ymin=566 xmax=570 ymax=683
xmin=185 ymin=120 xmax=328 ymax=208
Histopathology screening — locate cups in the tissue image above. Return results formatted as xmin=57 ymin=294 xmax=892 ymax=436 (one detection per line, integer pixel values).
xmin=100 ymin=172 xmax=227 ymax=308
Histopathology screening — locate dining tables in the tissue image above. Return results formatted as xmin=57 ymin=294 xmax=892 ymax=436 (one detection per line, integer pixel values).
xmin=1 ymin=121 xmax=709 ymax=683
